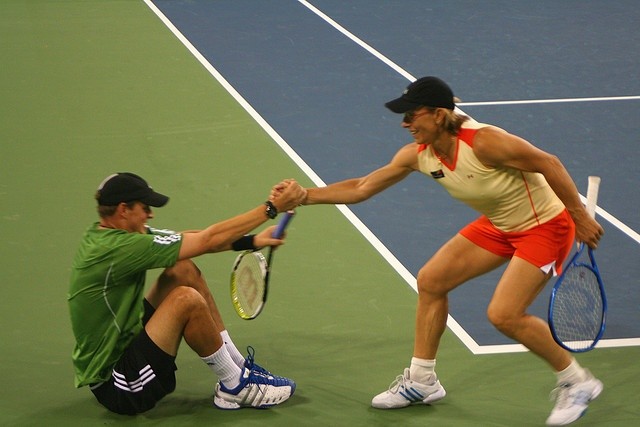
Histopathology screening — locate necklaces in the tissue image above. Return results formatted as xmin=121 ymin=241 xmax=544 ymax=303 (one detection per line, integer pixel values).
xmin=430 ymin=136 xmax=458 ymax=169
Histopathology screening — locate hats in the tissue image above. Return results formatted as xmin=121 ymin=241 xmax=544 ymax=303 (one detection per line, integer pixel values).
xmin=95 ymin=172 xmax=169 ymax=207
xmin=385 ymin=76 xmax=455 ymax=114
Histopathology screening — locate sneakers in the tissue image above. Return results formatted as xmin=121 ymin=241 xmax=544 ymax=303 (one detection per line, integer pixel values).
xmin=545 ymin=367 xmax=604 ymax=427
xmin=213 ymin=366 xmax=296 ymax=411
xmin=371 ymin=368 xmax=446 ymax=409
xmin=244 ymin=346 xmax=272 ymax=375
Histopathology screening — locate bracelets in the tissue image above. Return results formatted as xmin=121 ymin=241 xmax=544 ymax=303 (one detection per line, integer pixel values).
xmin=231 ymin=233 xmax=257 ymax=251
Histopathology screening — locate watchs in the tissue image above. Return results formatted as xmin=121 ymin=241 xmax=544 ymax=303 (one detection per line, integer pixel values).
xmin=265 ymin=200 xmax=277 ymax=219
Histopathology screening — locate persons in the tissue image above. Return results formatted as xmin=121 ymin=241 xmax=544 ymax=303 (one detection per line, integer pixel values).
xmin=65 ymin=172 xmax=296 ymax=416
xmin=269 ymin=76 xmax=606 ymax=427
